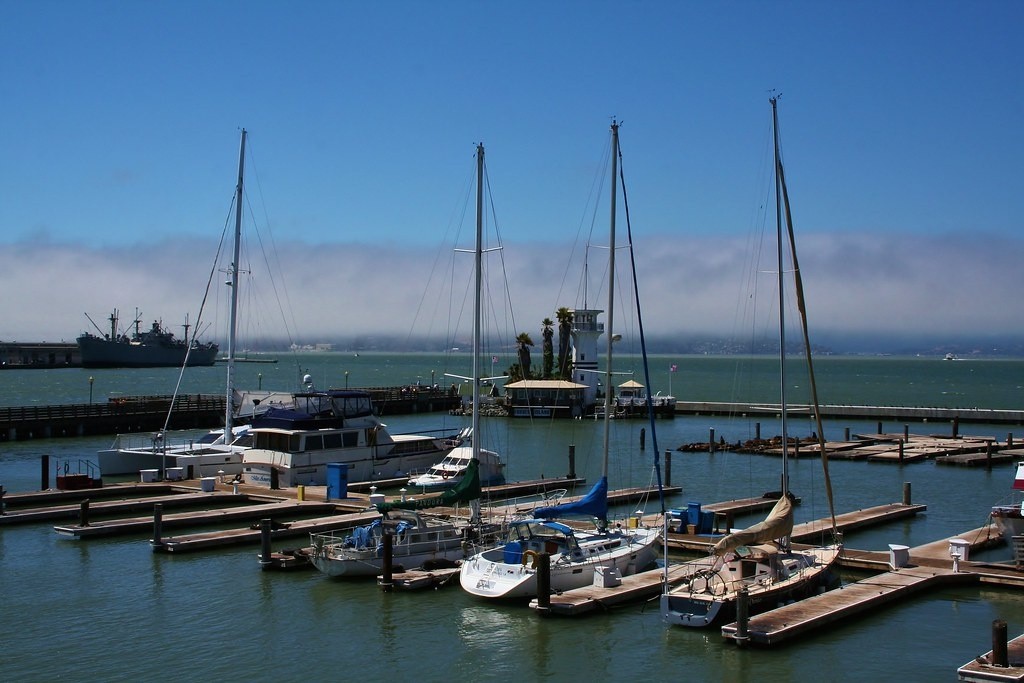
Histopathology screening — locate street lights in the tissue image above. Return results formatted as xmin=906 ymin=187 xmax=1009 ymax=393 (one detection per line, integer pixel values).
xmin=258 ymin=372 xmax=262 ymax=389
xmin=344 ymin=370 xmax=349 ymax=390
xmin=431 ymin=370 xmax=434 ymax=386
xmin=88 ymin=376 xmax=94 ymax=404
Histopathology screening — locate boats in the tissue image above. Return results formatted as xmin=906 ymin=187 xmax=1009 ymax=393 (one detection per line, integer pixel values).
xmin=988 ymin=458 xmax=1024 ymax=566
xmin=407 ymin=426 xmax=507 ymax=493
xmin=74 ymin=305 xmax=220 ymax=368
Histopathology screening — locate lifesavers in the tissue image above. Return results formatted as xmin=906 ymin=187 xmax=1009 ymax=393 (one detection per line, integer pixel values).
xmin=442 ymin=470 xmax=449 ymax=480
xmin=522 ymin=550 xmax=538 ymax=568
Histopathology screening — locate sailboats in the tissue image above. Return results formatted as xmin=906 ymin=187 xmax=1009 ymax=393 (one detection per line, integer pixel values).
xmin=97 ymin=123 xmax=477 ymax=491
xmin=459 ymin=114 xmax=673 ymax=602
xmin=655 ymin=86 xmax=848 ymax=630
xmin=303 ymin=140 xmax=550 ymax=577
